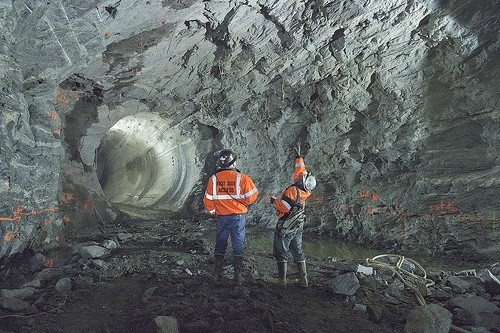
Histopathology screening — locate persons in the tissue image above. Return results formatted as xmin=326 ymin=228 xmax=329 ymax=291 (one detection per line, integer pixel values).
xmin=203 ymin=149 xmax=259 ymax=287
xmin=270 ymin=142 xmax=318 ymax=288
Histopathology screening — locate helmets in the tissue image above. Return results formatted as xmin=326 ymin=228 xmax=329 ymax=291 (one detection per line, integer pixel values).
xmin=218 ymin=148 xmax=237 ymax=167
xmin=303 ymin=171 xmax=316 ymax=191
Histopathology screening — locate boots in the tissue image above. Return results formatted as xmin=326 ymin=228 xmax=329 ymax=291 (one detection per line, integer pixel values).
xmin=214 ymin=254 xmax=224 ymax=286
xmin=231 ymin=255 xmax=243 ymax=286
xmin=294 ymin=259 xmax=308 ymax=287
xmin=271 ymin=260 xmax=287 ymax=288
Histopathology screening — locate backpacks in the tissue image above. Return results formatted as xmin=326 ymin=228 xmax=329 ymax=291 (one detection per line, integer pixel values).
xmin=277 ymin=185 xmax=306 ymax=237
